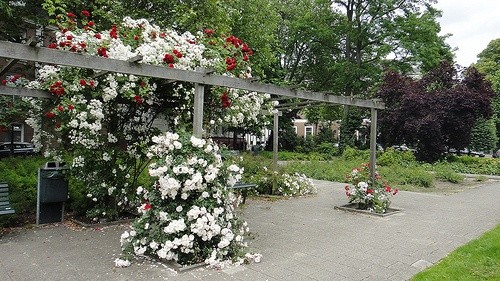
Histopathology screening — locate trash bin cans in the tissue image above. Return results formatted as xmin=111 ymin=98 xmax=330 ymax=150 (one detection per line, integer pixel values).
xmin=36 ymin=161 xmax=72 ymax=226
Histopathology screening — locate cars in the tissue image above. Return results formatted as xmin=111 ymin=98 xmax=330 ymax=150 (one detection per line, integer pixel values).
xmin=448 ymin=147 xmax=486 ymax=157
xmin=0 ymin=141 xmax=42 ymax=159
